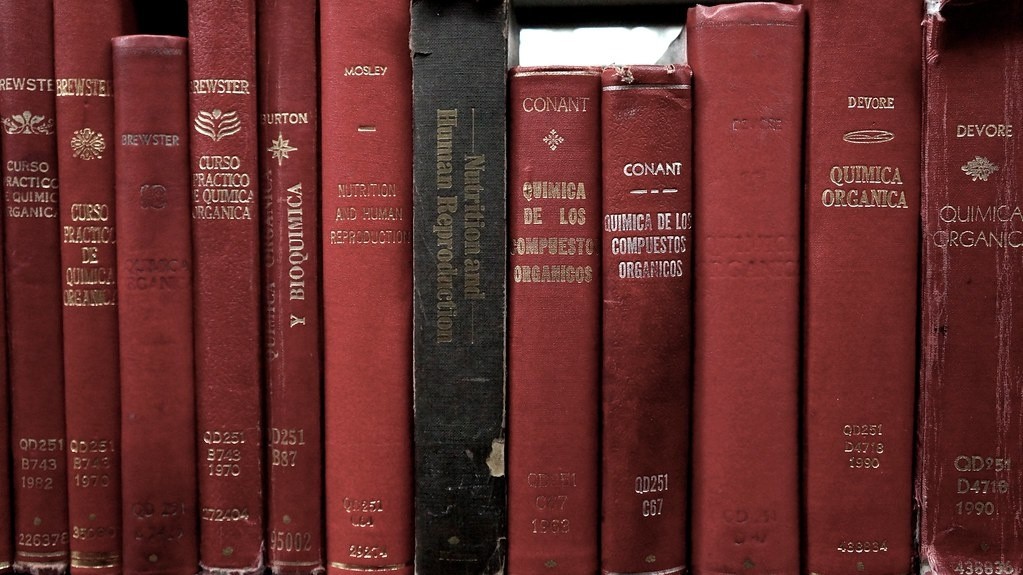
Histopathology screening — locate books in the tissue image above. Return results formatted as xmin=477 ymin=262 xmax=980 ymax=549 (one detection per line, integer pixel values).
xmin=2 ymin=0 xmax=1023 ymax=575
